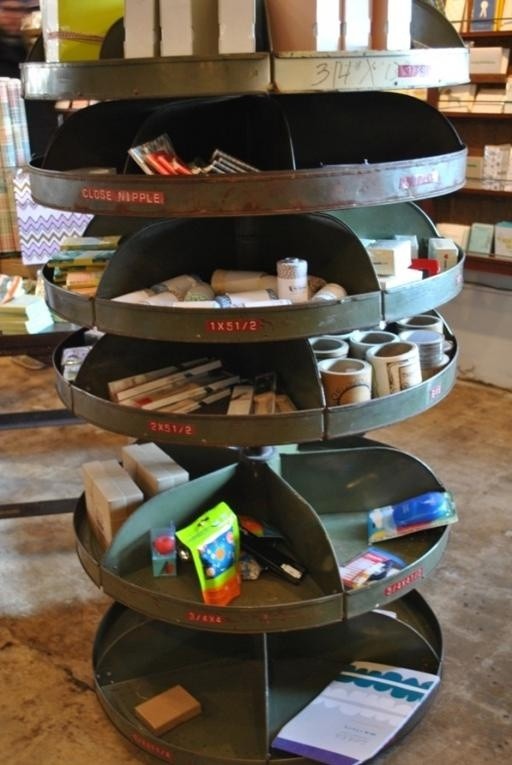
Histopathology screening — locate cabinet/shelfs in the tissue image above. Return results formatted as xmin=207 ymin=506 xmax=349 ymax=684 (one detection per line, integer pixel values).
xmin=395 ymin=0 xmax=510 ymax=402
xmin=19 ymin=0 xmax=470 ymax=763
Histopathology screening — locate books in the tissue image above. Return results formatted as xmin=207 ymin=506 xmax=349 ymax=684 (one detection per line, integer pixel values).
xmin=123 ymin=133 xmax=263 ymax=180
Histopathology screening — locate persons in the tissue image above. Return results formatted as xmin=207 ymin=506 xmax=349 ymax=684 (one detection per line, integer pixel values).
xmin=46 ymin=236 xmax=122 ymax=297
xmin=107 ymin=357 xmax=243 ymax=419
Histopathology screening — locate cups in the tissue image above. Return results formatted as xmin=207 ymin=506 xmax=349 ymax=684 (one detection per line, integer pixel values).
xmin=308 ymin=314 xmax=453 ymax=406
